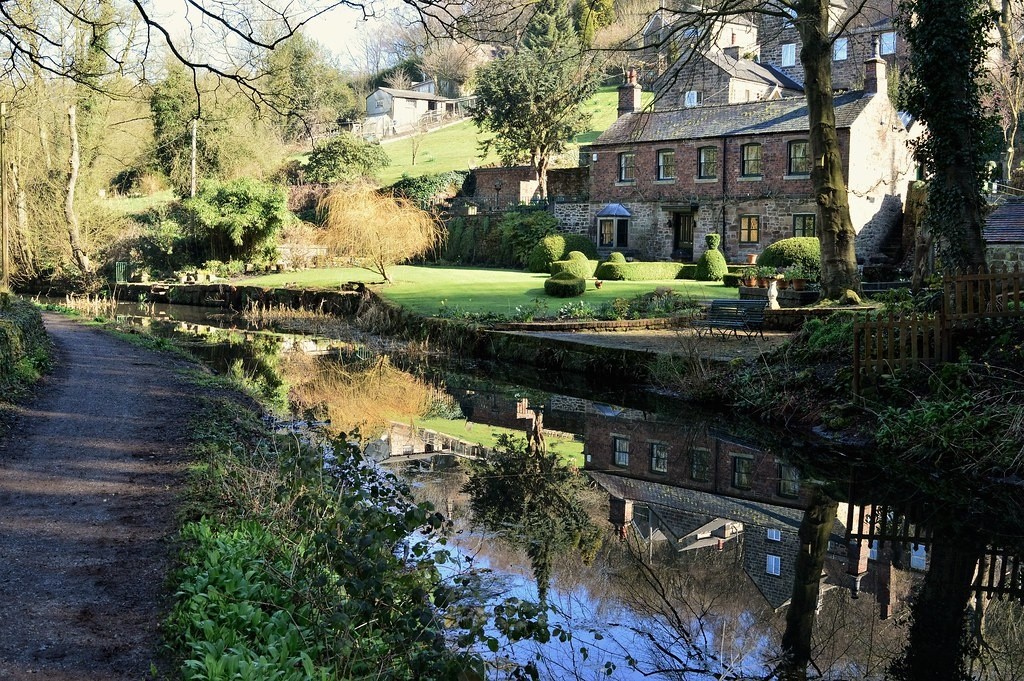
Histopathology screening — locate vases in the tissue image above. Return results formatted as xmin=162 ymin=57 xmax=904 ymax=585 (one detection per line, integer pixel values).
xmin=747 ymin=253 xmax=758 ymax=264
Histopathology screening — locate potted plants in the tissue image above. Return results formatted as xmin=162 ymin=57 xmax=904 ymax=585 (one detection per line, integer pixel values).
xmin=756 ymin=265 xmax=772 ymax=287
xmin=741 ymin=267 xmax=757 ymax=286
xmin=775 ymin=266 xmax=791 ymax=289
xmin=790 ymin=267 xmax=809 ymax=290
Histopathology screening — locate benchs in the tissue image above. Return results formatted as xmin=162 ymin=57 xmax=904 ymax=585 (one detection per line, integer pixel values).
xmin=689 ymin=299 xmax=770 ymax=342
